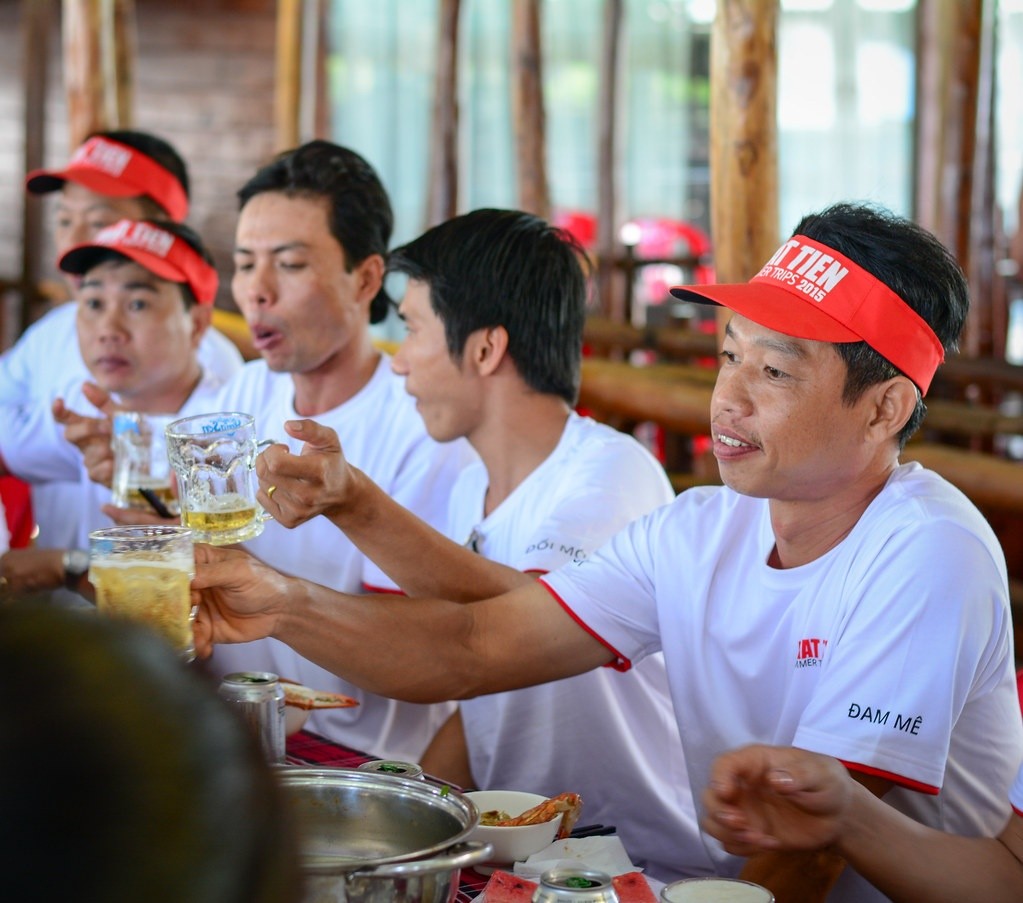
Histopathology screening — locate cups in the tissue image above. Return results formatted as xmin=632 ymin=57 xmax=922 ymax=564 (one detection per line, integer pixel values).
xmin=165 ymin=412 xmax=277 ymax=548
xmin=110 ymin=412 xmax=181 ymax=516
xmin=661 ymin=877 xmax=775 ymax=903
xmin=88 ymin=525 xmax=199 ymax=663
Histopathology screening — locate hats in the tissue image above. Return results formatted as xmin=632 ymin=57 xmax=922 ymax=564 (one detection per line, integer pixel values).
xmin=669 ymin=233 xmax=945 ymax=399
xmin=57 ymin=218 xmax=219 ymax=305
xmin=23 ymin=135 xmax=189 ymax=225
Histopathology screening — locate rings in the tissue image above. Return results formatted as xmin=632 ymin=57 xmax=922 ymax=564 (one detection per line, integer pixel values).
xmin=267 ymin=486 xmax=276 ymax=498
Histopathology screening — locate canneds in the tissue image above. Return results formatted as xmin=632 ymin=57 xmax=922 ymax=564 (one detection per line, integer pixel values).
xmin=218 ymin=671 xmax=287 ymax=768
xmin=357 ymin=760 xmax=425 ymax=780
xmin=531 ymin=866 xmax=621 ymax=903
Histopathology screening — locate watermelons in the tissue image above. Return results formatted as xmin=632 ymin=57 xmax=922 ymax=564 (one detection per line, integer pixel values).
xmin=610 ymin=871 xmax=659 ymax=903
xmin=480 ymin=869 xmax=538 ymax=903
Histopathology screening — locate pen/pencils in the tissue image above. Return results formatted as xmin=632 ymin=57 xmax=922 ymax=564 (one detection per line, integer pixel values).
xmin=139 ymin=487 xmax=173 ymax=519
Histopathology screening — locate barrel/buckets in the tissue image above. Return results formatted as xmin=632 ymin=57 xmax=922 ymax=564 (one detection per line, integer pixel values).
xmin=273 ymin=765 xmax=480 ymax=903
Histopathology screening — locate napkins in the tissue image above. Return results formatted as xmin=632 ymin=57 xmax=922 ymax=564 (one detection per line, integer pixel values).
xmin=513 ymin=835 xmax=645 ymax=882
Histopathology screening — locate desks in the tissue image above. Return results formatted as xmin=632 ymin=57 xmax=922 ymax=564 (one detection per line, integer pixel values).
xmin=575 ymin=315 xmax=1023 ymax=593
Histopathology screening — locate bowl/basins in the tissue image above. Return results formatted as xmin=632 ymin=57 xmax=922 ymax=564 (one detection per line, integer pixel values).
xmin=460 ymin=790 xmax=564 ymax=874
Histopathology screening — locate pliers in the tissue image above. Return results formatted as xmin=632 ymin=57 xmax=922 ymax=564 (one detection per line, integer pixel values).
xmin=553 ymin=824 xmax=617 ymax=842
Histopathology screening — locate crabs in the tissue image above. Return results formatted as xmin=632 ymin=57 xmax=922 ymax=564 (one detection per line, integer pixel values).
xmin=275 ymin=678 xmax=360 ymax=709
xmin=480 ymin=792 xmax=583 ymax=841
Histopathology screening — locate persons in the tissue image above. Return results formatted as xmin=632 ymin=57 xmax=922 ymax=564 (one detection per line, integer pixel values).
xmin=0 ymin=216 xmax=223 ymax=605
xmin=0 ymin=126 xmax=246 ymax=548
xmin=195 ymin=196 xmax=1023 ymax=902
xmin=699 ymin=743 xmax=1023 ymax=903
xmin=252 ymin=207 xmax=721 ymax=883
xmin=56 ymin=138 xmax=482 ymax=765
xmin=0 ymin=593 xmax=285 ymax=903
